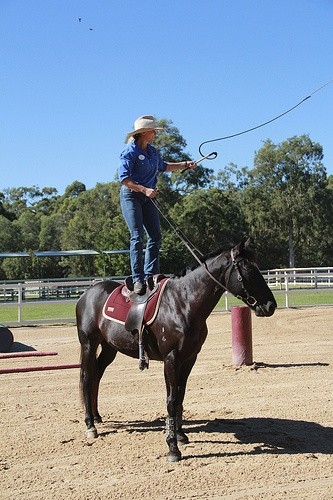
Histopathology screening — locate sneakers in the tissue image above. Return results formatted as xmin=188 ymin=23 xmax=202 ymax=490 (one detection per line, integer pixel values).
xmin=146 ymin=277 xmax=155 ymax=286
xmin=133 ymin=281 xmax=146 ymax=294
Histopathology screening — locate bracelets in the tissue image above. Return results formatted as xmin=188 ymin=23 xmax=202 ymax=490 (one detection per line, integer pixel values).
xmin=183 ymin=160 xmax=187 ymax=168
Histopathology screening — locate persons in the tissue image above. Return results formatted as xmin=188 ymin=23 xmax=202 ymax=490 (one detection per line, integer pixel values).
xmin=118 ymin=114 xmax=197 ymax=294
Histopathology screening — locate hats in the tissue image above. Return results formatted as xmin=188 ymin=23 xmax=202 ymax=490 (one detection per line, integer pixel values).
xmin=126 ymin=115 xmax=163 ymax=136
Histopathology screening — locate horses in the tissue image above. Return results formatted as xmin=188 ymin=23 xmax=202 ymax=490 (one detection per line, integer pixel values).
xmin=75 ymin=237 xmax=277 ymax=462
